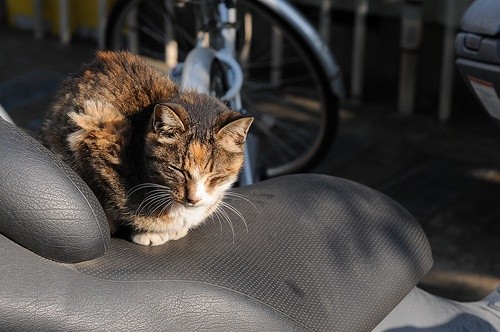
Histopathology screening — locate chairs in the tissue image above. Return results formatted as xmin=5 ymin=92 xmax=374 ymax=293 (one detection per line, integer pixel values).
xmin=1 ymin=118 xmax=434 ymax=332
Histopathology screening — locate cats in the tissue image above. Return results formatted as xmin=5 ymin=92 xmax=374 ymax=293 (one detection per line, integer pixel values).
xmin=42 ymin=51 xmax=255 ymax=246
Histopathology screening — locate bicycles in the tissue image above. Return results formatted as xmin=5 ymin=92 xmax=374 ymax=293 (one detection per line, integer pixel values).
xmin=104 ymin=0 xmax=345 ymax=186
